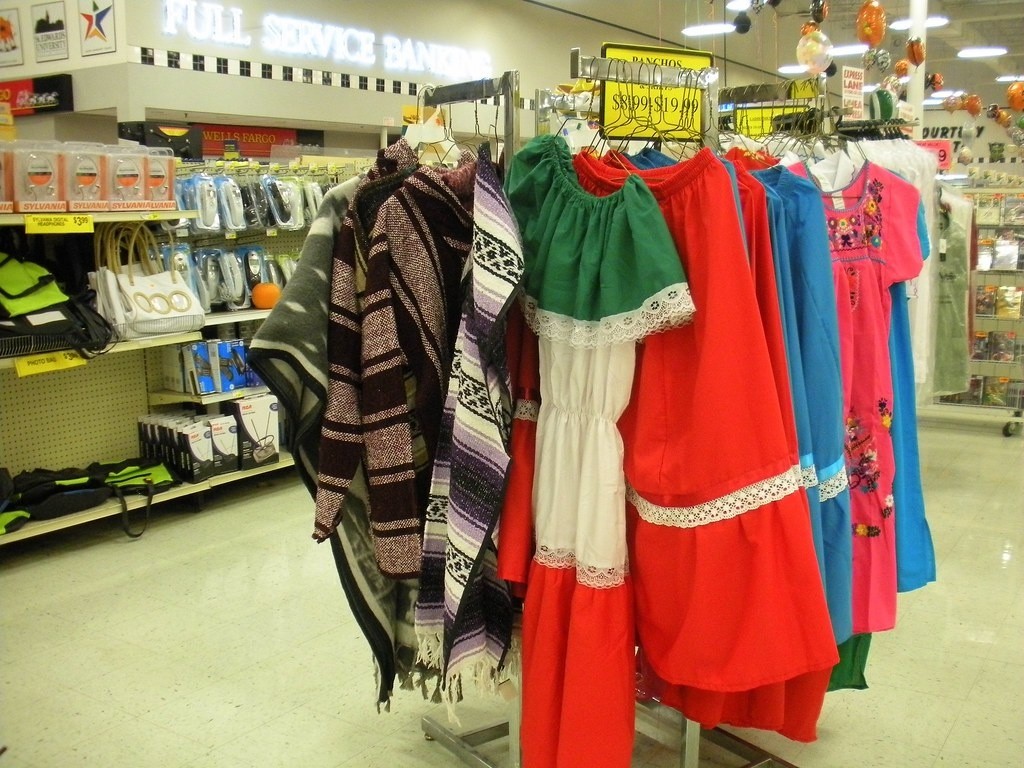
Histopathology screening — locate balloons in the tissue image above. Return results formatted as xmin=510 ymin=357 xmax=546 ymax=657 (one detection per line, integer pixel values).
xmin=958 ymin=124 xmax=976 ymax=165
xmin=856 ymin=0 xmax=944 ymax=120
xmin=943 ymin=93 xmax=982 ymax=118
xmin=987 ymin=80 xmax=1024 ymax=158
xmin=733 ymin=0 xmax=782 ymax=34
xmin=796 ymin=0 xmax=837 ymax=77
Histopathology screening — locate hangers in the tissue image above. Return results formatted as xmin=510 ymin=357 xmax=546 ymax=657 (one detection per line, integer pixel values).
xmin=407 ymin=56 xmax=910 ymax=177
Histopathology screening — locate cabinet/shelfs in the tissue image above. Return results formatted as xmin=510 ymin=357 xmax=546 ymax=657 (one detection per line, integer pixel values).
xmin=149 ymin=165 xmax=311 ymax=487
xmin=0 ymin=209 xmax=212 ymax=544
xmin=915 ymin=188 xmax=1024 ymax=436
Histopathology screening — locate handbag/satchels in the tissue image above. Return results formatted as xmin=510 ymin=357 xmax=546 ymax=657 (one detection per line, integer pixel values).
xmin=86 ymin=219 xmax=206 ymax=341
xmin=0 ymin=246 xmax=119 ymax=360
xmin=0 ymin=467 xmax=31 ymax=535
xmin=14 ymin=466 xmax=115 ymax=521
xmin=84 ymin=456 xmax=183 ymax=538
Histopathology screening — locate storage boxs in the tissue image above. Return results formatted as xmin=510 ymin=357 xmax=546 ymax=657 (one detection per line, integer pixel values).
xmin=137 ymin=336 xmax=281 ymax=484
